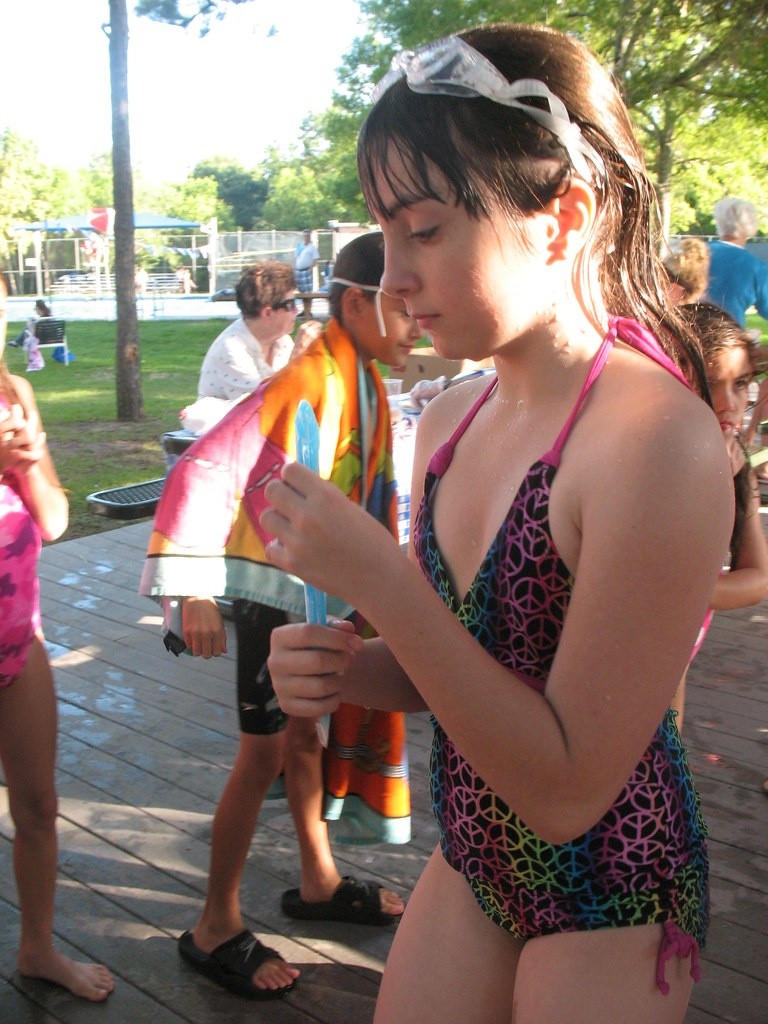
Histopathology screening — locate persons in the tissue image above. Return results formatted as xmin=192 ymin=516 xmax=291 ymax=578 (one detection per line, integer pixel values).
xmin=0 ymin=231 xmax=420 ymax=1000
xmin=257 ymin=23 xmax=734 ymax=1024
xmin=655 ymin=194 xmax=768 ymax=733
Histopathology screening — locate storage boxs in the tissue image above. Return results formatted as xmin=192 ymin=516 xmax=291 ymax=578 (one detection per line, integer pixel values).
xmin=389 ymin=347 xmax=494 ymax=396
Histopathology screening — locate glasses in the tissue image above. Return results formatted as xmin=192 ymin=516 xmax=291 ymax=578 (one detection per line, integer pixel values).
xmin=271 ymin=297 xmax=304 ymax=312
xmin=659 ymin=262 xmax=694 ymax=296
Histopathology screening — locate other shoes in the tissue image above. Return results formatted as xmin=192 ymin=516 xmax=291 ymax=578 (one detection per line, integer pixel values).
xmin=296 ymin=312 xmax=313 ymax=317
xmin=7 ymin=340 xmax=18 ymax=347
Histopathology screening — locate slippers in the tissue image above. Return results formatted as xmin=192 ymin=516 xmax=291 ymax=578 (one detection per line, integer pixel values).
xmin=178 ymin=927 xmax=297 ymax=1001
xmin=282 ymin=876 xmax=407 ymax=927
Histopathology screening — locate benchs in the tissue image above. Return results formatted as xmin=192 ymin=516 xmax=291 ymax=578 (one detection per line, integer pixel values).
xmin=45 ymin=273 xmax=186 ymax=294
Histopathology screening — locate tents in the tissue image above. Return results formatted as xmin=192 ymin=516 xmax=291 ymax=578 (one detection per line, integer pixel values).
xmin=19 ymin=206 xmax=200 ymax=299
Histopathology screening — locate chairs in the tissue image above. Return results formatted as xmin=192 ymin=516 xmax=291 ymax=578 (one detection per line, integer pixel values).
xmin=24 ymin=319 xmax=68 ymax=367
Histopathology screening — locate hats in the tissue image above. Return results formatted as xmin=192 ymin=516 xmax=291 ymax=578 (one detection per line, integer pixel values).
xmin=302 ymin=229 xmax=311 ymax=236
